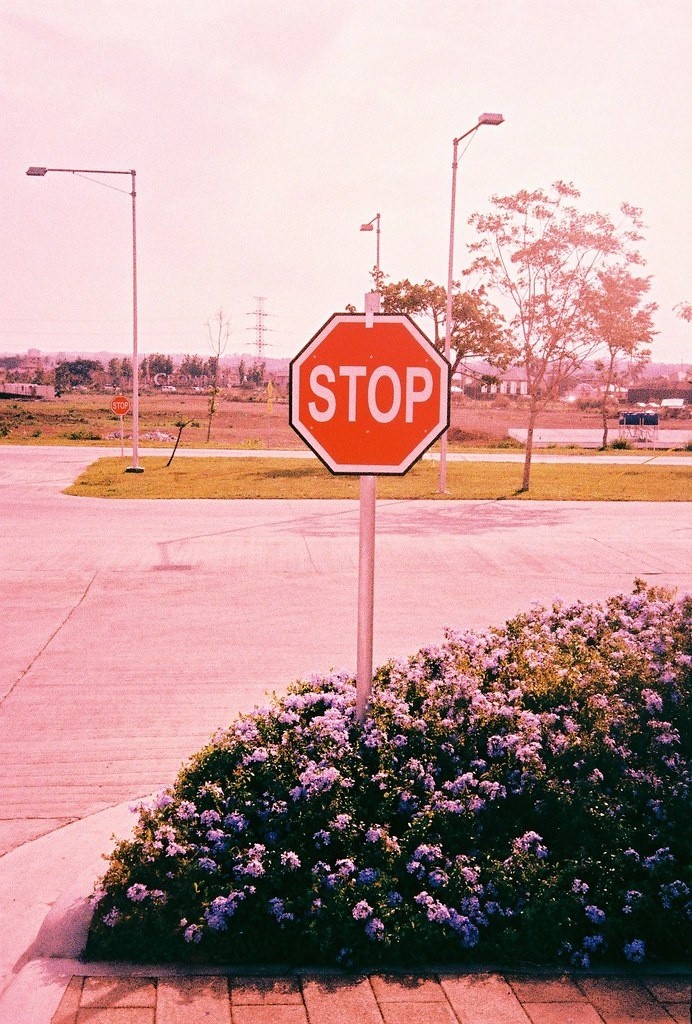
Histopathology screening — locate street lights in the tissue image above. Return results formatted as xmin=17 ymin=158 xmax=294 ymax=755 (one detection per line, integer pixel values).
xmin=432 ymin=111 xmax=507 ymax=500
xmin=359 ymin=214 xmax=381 ymax=294
xmin=25 ymin=166 xmax=146 ymax=476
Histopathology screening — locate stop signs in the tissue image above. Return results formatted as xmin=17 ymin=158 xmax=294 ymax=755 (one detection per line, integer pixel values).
xmin=110 ymin=395 xmax=131 ymax=417
xmin=288 ymin=310 xmax=452 ymax=479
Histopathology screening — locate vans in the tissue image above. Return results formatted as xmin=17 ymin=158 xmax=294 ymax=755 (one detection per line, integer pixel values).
xmin=161 ymin=386 xmax=177 ymax=392
